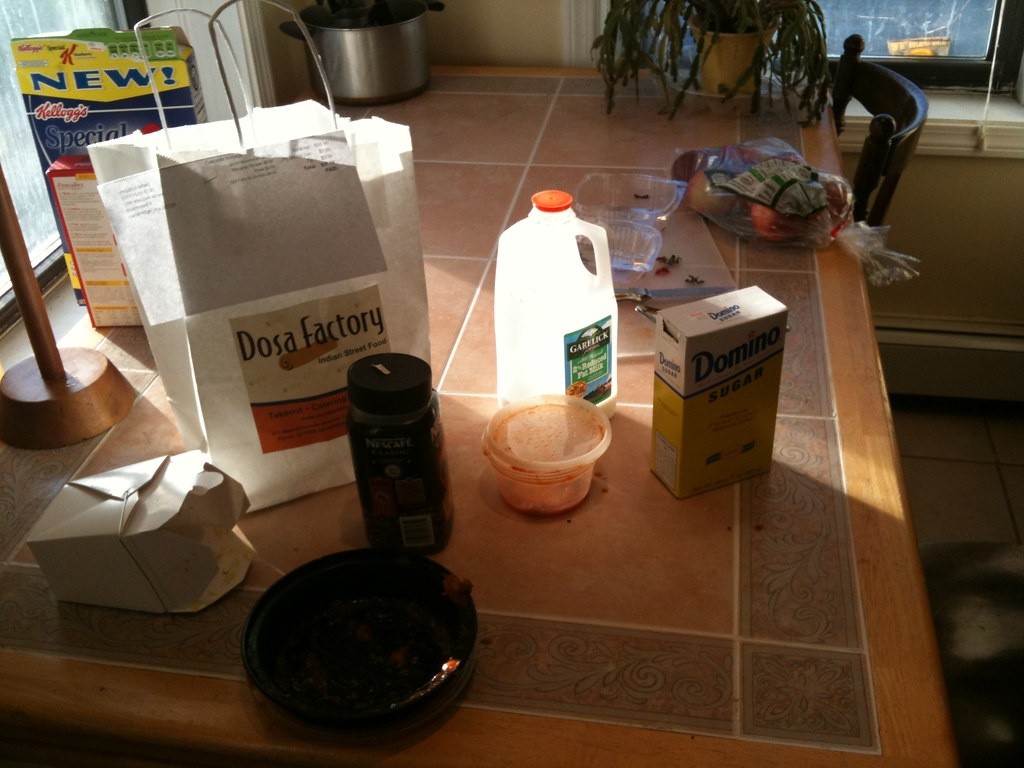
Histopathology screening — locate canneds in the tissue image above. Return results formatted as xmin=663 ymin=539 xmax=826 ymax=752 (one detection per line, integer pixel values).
xmin=345 ymin=352 xmax=455 ymax=556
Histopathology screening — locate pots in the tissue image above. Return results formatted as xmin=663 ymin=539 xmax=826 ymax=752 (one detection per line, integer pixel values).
xmin=279 ymin=0 xmax=444 ymax=104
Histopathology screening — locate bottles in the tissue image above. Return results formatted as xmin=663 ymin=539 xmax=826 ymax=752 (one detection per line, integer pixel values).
xmin=346 ymin=352 xmax=453 ymax=552
xmin=494 ymin=189 xmax=618 ymax=421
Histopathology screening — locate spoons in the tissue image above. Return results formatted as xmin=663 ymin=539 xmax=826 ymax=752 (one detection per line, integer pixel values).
xmin=634 ymin=306 xmax=790 ymax=334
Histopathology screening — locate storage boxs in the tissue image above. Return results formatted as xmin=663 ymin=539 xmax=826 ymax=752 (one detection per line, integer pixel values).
xmin=45 ymin=155 xmax=142 ymax=329
xmin=7 ymin=26 xmax=209 ymax=308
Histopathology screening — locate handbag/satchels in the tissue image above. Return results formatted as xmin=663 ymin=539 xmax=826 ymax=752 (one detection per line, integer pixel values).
xmin=85 ymin=0 xmax=432 ymax=513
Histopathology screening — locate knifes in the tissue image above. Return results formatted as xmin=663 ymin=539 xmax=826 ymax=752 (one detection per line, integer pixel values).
xmin=615 ymin=283 xmax=734 ymax=302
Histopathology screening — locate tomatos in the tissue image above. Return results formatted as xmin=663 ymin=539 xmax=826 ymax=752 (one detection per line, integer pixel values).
xmin=684 ymin=137 xmax=850 ymax=241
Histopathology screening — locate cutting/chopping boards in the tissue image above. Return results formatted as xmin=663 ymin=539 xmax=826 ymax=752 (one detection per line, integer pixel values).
xmin=577 ymin=211 xmax=737 ymax=358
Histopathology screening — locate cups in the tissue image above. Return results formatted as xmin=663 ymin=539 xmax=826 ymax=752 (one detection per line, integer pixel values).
xmin=483 ymin=396 xmax=612 ymax=516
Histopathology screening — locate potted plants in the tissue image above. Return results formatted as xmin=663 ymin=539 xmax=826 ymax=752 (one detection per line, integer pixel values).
xmin=589 ymin=0 xmax=829 ymax=128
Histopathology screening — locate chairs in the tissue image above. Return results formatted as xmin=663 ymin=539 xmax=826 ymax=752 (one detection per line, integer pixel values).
xmin=828 ymin=34 xmax=929 ymax=227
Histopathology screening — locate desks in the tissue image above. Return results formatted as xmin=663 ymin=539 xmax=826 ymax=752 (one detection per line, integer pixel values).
xmin=1 ymin=58 xmax=955 ymax=766
